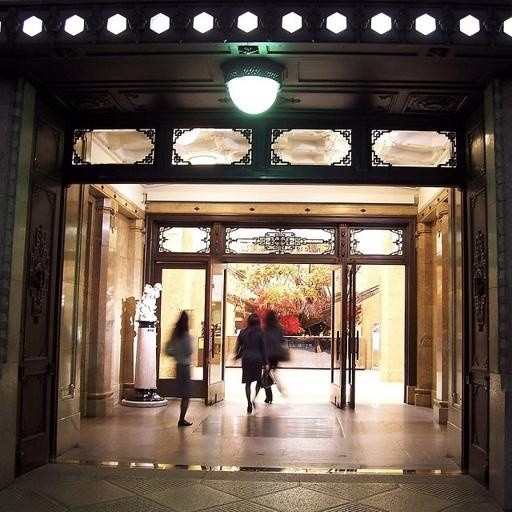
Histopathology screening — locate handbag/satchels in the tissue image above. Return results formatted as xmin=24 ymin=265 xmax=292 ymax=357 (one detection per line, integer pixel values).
xmin=166 ymin=340 xmax=176 ymax=355
xmin=262 ymin=368 xmax=273 ymax=388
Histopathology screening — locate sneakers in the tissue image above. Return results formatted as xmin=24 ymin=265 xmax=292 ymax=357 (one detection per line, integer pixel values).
xmin=246 ymin=402 xmax=252 ymax=413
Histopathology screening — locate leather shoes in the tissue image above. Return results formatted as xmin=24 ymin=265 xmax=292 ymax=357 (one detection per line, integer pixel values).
xmin=179 ymin=419 xmax=192 ymax=426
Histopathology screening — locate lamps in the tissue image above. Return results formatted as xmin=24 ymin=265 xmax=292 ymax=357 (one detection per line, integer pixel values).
xmin=219 ymin=57 xmax=288 ymax=115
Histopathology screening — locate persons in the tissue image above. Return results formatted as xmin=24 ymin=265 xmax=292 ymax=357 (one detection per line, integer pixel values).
xmin=233 ymin=311 xmax=272 ymax=416
xmin=163 ymin=310 xmax=197 ymax=428
xmin=261 ymin=308 xmax=291 ymax=405
